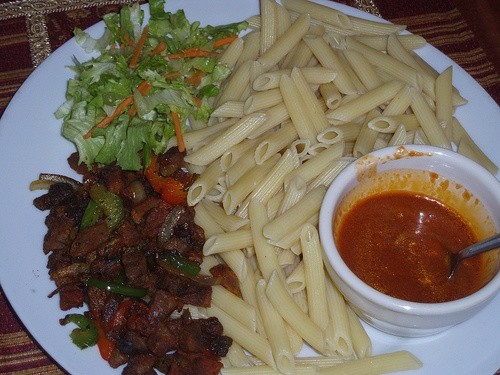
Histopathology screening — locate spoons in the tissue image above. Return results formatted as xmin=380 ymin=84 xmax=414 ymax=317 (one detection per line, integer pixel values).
xmin=447 ymin=234 xmax=500 ymax=281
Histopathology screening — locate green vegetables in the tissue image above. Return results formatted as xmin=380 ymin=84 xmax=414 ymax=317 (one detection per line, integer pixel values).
xmin=53 ymin=0 xmax=249 ymax=168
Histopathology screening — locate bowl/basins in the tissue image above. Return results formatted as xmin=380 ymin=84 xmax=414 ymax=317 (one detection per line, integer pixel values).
xmin=318 ymin=145 xmax=500 ymax=338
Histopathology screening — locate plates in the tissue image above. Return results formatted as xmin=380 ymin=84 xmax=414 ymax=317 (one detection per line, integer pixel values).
xmin=0 ymin=0 xmax=500 ymax=375
